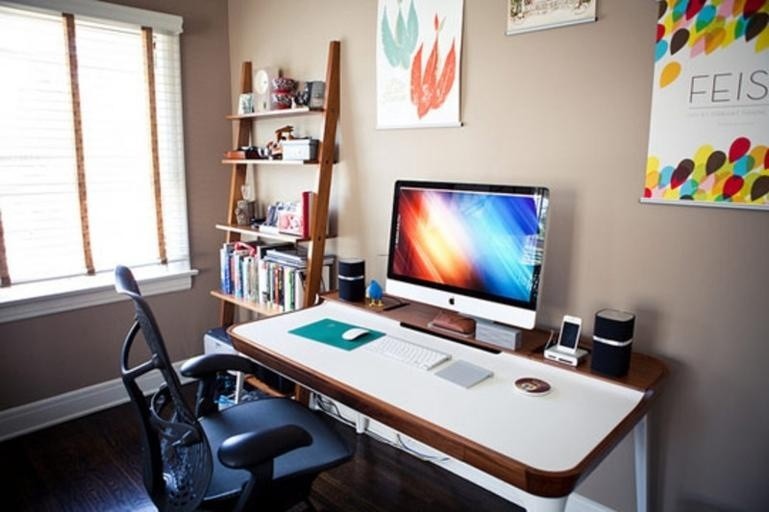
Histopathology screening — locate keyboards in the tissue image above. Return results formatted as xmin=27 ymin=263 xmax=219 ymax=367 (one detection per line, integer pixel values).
xmin=362 ymin=334 xmax=450 ymax=373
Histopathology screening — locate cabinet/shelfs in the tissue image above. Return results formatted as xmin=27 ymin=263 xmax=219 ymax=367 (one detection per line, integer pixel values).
xmin=208 ymin=39 xmax=341 ymax=405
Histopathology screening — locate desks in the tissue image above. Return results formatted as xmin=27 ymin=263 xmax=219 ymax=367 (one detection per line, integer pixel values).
xmin=225 ymin=288 xmax=672 ymax=512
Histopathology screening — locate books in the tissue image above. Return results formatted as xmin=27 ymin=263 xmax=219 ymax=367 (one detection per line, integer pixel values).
xmin=217 ymin=236 xmax=336 ymax=313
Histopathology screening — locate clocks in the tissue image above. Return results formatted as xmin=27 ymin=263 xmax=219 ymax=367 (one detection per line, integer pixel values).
xmin=252 ymin=65 xmax=279 ymax=113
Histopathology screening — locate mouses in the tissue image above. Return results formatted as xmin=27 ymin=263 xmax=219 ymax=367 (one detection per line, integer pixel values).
xmin=342 ymin=326 xmax=369 ymax=341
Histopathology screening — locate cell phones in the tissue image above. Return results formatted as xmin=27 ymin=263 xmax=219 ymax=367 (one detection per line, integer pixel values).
xmin=556 ymin=315 xmax=584 ymax=354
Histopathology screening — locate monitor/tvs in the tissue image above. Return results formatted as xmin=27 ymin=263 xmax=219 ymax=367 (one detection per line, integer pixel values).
xmin=384 ymin=178 xmax=551 ymax=339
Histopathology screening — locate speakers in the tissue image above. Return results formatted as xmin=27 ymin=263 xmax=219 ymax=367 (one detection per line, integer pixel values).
xmin=338 ymin=255 xmax=366 ymax=305
xmin=591 ymin=308 xmax=637 ymax=378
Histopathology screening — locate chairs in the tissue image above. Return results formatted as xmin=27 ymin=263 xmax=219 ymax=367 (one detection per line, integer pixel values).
xmin=112 ymin=265 xmax=355 ymax=512
